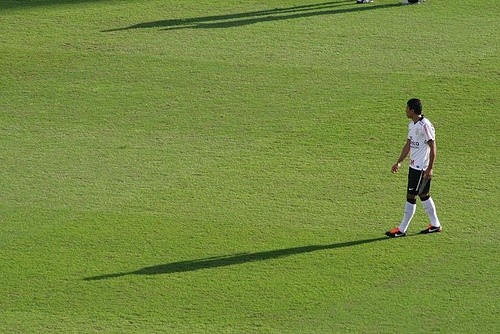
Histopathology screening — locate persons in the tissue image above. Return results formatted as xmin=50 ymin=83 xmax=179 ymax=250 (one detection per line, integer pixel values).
xmin=381 ymin=98 xmax=443 ymax=239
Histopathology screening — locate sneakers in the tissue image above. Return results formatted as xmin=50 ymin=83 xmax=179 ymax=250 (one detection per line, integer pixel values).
xmin=385 ymin=228 xmax=406 ymax=238
xmin=420 ymin=225 xmax=443 ymax=233
